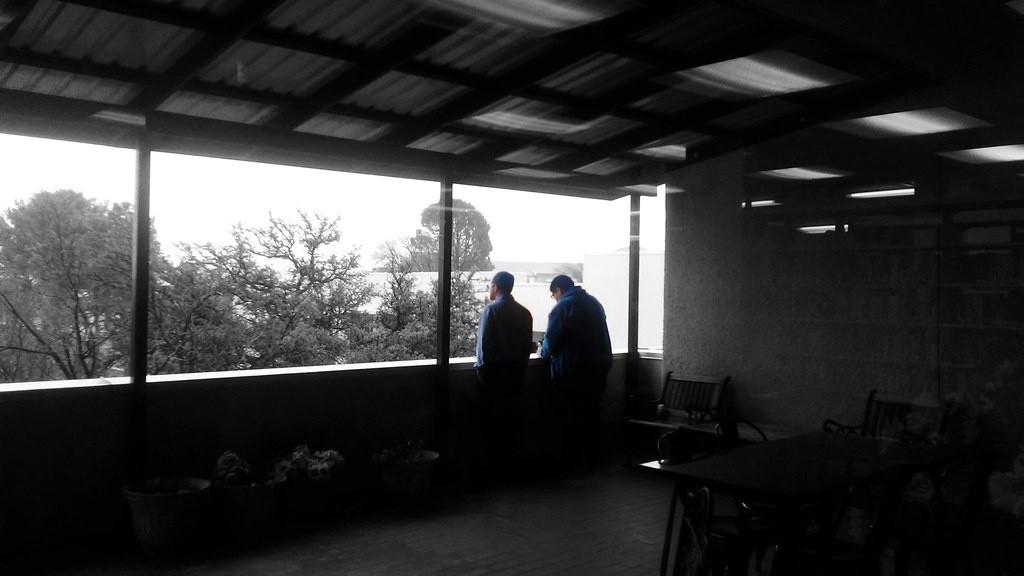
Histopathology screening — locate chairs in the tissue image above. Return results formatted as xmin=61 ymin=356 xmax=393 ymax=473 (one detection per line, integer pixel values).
xmin=620 ymin=369 xmax=727 ymax=473
xmin=823 ymin=388 xmax=963 ymax=514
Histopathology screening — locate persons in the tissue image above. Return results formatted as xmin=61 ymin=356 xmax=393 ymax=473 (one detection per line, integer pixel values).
xmin=473 ymin=270 xmax=534 ymax=470
xmin=539 ymin=274 xmax=614 ymax=469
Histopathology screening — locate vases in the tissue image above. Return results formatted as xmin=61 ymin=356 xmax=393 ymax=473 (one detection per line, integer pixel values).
xmin=215 ymin=465 xmax=294 ymax=544
xmin=373 ymin=445 xmax=444 ymax=518
xmin=121 ymin=476 xmax=212 ymax=563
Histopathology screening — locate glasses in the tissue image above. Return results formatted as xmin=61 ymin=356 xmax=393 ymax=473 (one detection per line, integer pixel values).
xmin=486 ymin=283 xmax=495 ymax=288
xmin=551 ymin=293 xmax=556 ymax=300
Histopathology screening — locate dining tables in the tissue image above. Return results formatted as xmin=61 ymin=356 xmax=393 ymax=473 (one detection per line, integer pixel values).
xmin=633 ymin=429 xmax=964 ymax=576
